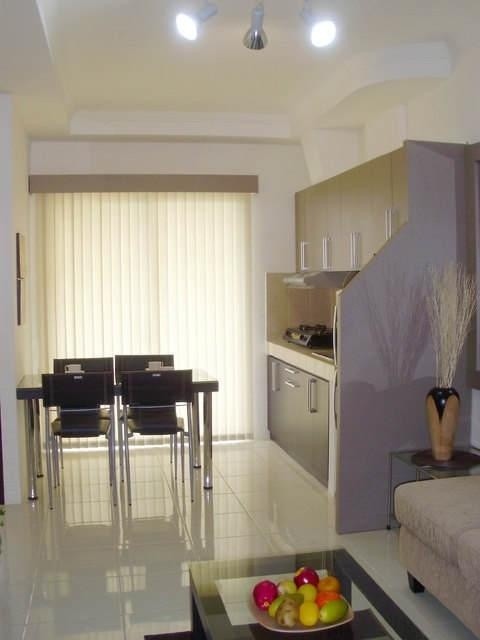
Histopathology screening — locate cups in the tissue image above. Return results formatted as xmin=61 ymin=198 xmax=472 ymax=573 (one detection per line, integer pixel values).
xmin=149 ymin=361 xmax=162 ymax=370
xmin=65 ymin=364 xmax=81 ymax=373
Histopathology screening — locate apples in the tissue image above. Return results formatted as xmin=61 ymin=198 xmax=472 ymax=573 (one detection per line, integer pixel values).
xmin=277 ymin=580 xmax=297 ymax=594
xmin=317 ymin=576 xmax=340 ymax=592
xmin=317 ymin=592 xmax=338 ymax=609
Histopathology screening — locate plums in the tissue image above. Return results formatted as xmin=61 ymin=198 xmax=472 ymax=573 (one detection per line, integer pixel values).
xmin=293 ymin=567 xmax=319 ymax=588
xmin=254 ymin=580 xmax=277 ymax=610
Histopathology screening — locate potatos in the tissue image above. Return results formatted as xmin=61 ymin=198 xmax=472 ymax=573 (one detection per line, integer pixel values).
xmin=276 ymin=599 xmax=299 ymax=628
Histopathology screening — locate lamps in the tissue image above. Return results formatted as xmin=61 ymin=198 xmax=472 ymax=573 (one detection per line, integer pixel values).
xmin=172 ymin=2 xmax=344 ymax=54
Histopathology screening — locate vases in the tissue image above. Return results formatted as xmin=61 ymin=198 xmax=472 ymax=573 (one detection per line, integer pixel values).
xmin=423 ymin=383 xmax=465 ymax=463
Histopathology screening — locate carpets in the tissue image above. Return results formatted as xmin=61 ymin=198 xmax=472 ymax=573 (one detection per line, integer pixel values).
xmin=144 ymin=607 xmax=395 ymax=639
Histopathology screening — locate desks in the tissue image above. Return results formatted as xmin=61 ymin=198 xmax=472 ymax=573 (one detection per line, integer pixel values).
xmin=11 ymin=372 xmax=222 ymax=495
xmin=183 ymin=544 xmax=429 ymax=640
xmin=385 ymin=444 xmax=478 ymax=535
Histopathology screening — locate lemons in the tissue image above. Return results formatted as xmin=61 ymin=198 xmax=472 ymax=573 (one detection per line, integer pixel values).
xmin=299 ymin=602 xmax=319 ymax=626
xmin=268 ymin=594 xmax=304 ymax=617
xmin=319 ymin=599 xmax=347 ymax=624
xmin=297 ymin=584 xmax=317 ymax=602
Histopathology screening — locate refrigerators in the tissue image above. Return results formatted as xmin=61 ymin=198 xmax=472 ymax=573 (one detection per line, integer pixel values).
xmin=331 ymin=290 xmax=341 ymax=499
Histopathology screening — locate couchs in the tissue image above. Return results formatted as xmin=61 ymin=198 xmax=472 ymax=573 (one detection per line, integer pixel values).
xmin=388 ymin=470 xmax=480 ymax=640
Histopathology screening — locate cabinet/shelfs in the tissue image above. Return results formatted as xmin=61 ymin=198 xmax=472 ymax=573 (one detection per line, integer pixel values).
xmin=264 ymin=355 xmax=330 ymax=488
xmin=291 ymin=143 xmax=474 ymax=278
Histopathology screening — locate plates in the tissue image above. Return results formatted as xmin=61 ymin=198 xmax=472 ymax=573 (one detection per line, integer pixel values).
xmin=250 ymin=581 xmax=354 ymax=635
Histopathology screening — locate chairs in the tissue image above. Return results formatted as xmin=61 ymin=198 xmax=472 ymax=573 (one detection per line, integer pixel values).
xmin=118 ymin=367 xmax=200 ymax=510
xmin=112 ymin=352 xmax=179 ymax=484
xmin=38 ymin=372 xmax=124 ymax=513
xmin=48 ymin=356 xmax=123 ymax=487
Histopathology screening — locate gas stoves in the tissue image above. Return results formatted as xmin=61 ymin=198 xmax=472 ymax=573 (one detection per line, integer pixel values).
xmin=284 ymin=322 xmax=335 ymax=350
